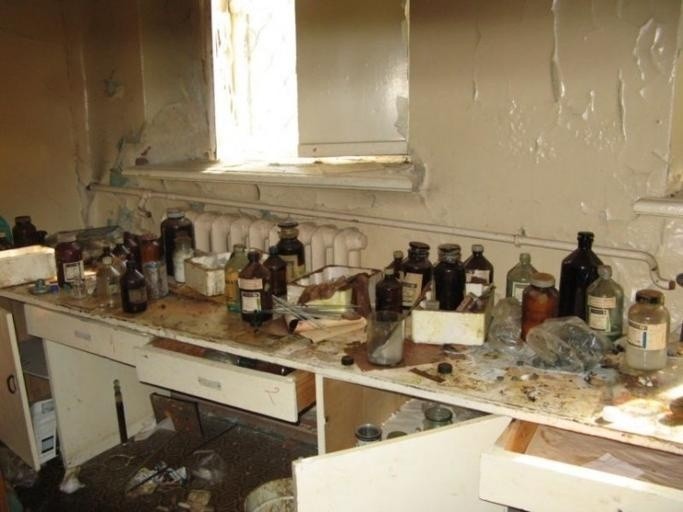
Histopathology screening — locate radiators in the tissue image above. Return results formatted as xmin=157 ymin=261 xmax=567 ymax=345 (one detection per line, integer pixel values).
xmin=157 ymin=207 xmax=368 ymax=274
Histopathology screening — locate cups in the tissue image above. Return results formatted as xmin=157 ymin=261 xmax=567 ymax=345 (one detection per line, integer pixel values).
xmin=365 ymin=309 xmax=406 ymax=366
xmin=72 ymin=278 xmax=89 ymax=300
xmin=104 ymin=285 xmax=124 ymax=313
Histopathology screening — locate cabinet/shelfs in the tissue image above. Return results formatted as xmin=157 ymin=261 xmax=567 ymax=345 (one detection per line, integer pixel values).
xmin=0 ymin=275 xmax=682 ymax=512
